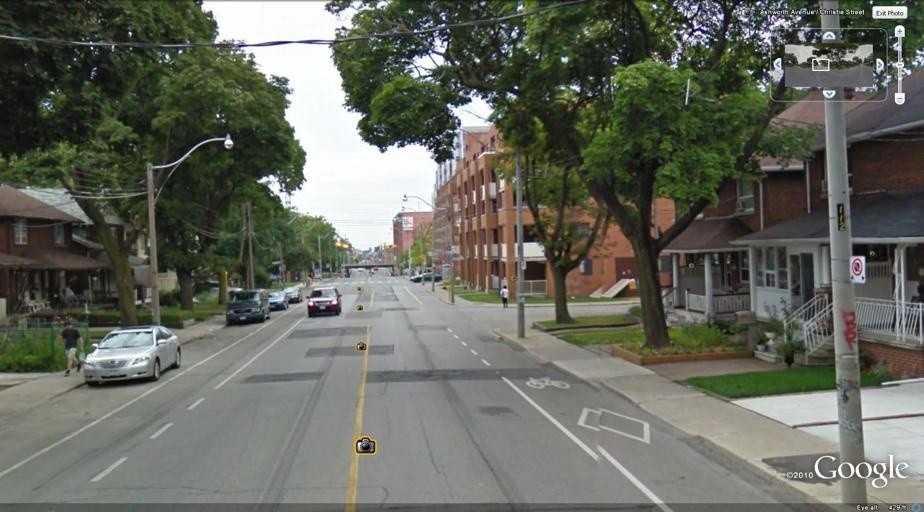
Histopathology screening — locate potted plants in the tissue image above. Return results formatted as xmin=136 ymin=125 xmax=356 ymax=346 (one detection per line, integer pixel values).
xmin=761 ymin=319 xmax=777 ymax=345
xmin=753 ymin=331 xmax=768 ymax=352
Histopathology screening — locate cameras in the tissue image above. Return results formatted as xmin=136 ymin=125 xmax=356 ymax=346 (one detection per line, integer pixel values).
xmin=355 ymin=437 xmax=376 ymax=454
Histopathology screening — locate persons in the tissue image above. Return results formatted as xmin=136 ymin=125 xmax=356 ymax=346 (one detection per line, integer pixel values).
xmin=499 ymin=285 xmax=509 ymax=309
xmin=910 ymin=284 xmax=924 ymax=304
xmin=56 ymin=320 xmax=84 ymax=377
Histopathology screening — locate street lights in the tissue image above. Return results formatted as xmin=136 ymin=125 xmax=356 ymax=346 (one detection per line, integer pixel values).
xmin=477 ymin=144 xmax=527 ymax=339
xmin=142 ymin=132 xmax=235 ymax=326
xmin=402 ymin=194 xmax=455 ymax=303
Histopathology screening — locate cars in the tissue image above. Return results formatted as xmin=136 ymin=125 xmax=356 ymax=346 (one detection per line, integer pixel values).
xmin=77 ymin=325 xmax=187 ymax=386
xmin=373 ymin=268 xmax=378 ymax=271
xmin=409 ymin=272 xmax=442 ymax=283
xmin=207 ymin=280 xmax=342 ymax=326
xmin=357 ymin=267 xmax=364 ymax=273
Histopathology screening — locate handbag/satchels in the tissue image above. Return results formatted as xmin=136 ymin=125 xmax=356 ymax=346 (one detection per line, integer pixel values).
xmin=501 ymin=289 xmax=504 ymax=297
